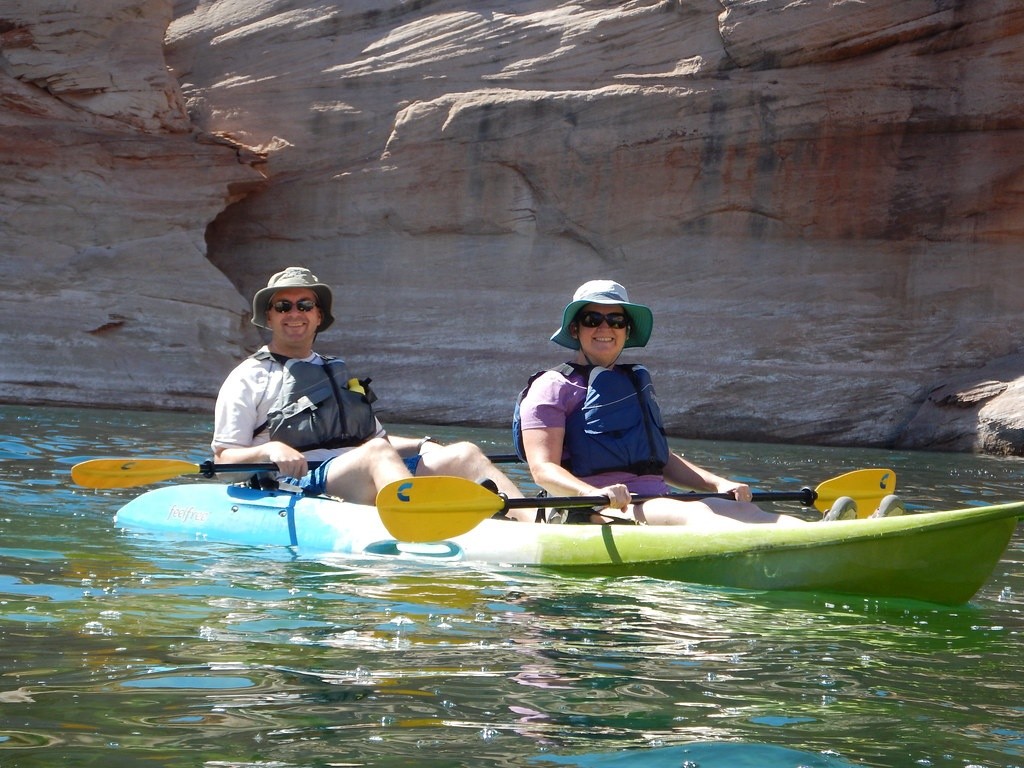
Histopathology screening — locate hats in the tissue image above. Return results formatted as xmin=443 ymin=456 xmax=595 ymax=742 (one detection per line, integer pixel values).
xmin=549 ymin=279 xmax=653 ymax=351
xmin=251 ymin=267 xmax=335 ymax=331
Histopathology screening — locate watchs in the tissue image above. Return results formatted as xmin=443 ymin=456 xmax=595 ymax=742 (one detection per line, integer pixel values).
xmin=416 ymin=436 xmax=431 ymax=454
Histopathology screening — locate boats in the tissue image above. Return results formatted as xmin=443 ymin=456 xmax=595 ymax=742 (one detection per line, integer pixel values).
xmin=115 ymin=470 xmax=1024 ymax=607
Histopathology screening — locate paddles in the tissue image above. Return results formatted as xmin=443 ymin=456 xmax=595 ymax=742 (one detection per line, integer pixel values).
xmin=372 ymin=466 xmax=900 ymax=548
xmin=65 ymin=450 xmax=523 ymax=494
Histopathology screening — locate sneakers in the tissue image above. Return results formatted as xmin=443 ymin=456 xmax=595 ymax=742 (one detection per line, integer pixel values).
xmin=868 ymin=494 xmax=904 ymax=518
xmin=821 ymin=495 xmax=857 ymax=520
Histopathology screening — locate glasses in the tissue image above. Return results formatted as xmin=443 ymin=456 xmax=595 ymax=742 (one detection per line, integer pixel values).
xmin=270 ymin=298 xmax=321 ymax=312
xmin=576 ymin=312 xmax=627 ymax=329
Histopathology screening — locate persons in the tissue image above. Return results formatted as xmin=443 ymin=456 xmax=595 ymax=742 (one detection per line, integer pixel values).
xmin=210 ymin=266 xmax=544 ymax=523
xmin=518 ymin=279 xmax=907 ymax=528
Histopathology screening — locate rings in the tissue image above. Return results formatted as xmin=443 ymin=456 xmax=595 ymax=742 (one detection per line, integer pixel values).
xmin=747 ymin=495 xmax=751 ymax=497
